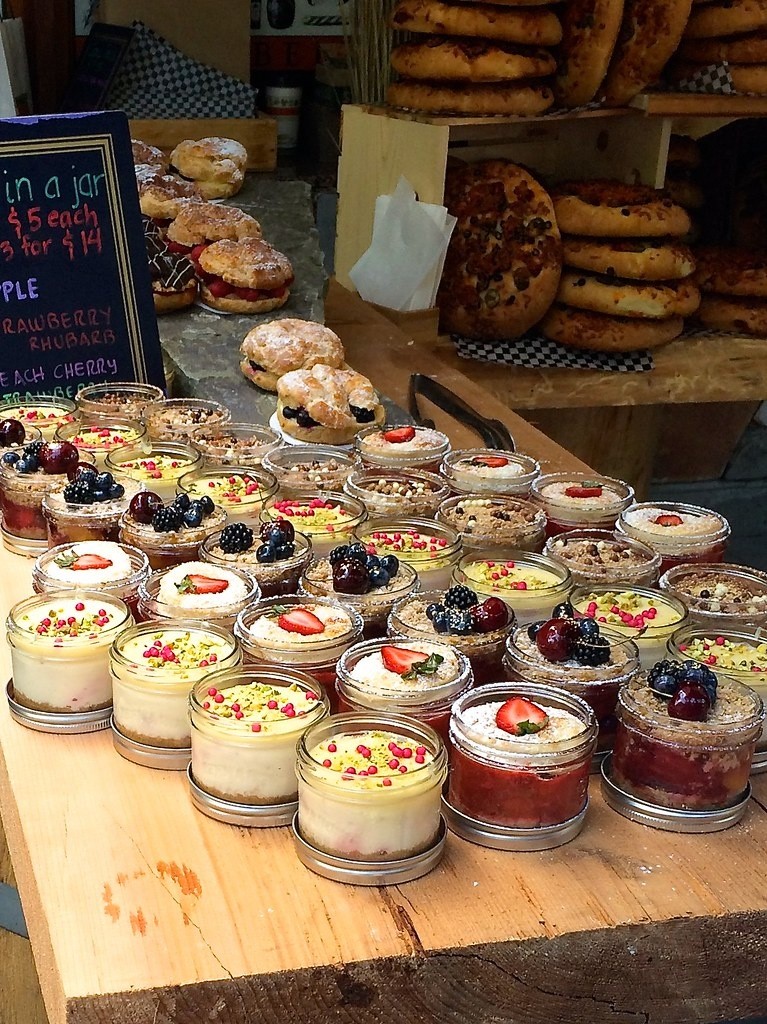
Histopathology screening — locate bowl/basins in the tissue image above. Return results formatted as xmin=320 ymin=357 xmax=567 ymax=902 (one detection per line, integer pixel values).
xmin=1 ymin=382 xmax=767 ymax=883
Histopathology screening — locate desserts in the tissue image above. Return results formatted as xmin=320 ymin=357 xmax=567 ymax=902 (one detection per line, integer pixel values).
xmin=0 ymin=378 xmax=766 ymax=863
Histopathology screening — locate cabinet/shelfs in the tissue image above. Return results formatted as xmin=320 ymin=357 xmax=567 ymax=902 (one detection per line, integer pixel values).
xmin=336 ymin=101 xmax=766 ymax=311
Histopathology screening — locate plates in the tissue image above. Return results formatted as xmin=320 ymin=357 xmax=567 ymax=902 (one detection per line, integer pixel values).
xmin=268 ymin=411 xmax=353 ymax=449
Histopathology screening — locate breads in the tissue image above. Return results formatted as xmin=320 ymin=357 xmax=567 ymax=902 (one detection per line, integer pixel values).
xmin=240 ymin=317 xmax=344 ymax=394
xmin=276 ymin=364 xmax=387 ymax=447
xmin=434 ymin=113 xmax=767 ymax=350
xmin=131 ymin=138 xmax=294 ymax=313
xmin=379 ymin=0 xmax=767 ymax=113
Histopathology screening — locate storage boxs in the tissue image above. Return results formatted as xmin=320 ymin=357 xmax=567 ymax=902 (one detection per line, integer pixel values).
xmin=80 ymin=0 xmax=278 ymax=174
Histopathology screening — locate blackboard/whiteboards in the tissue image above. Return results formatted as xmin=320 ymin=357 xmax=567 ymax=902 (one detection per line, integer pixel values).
xmin=0 ymin=112 xmax=165 ymax=404
